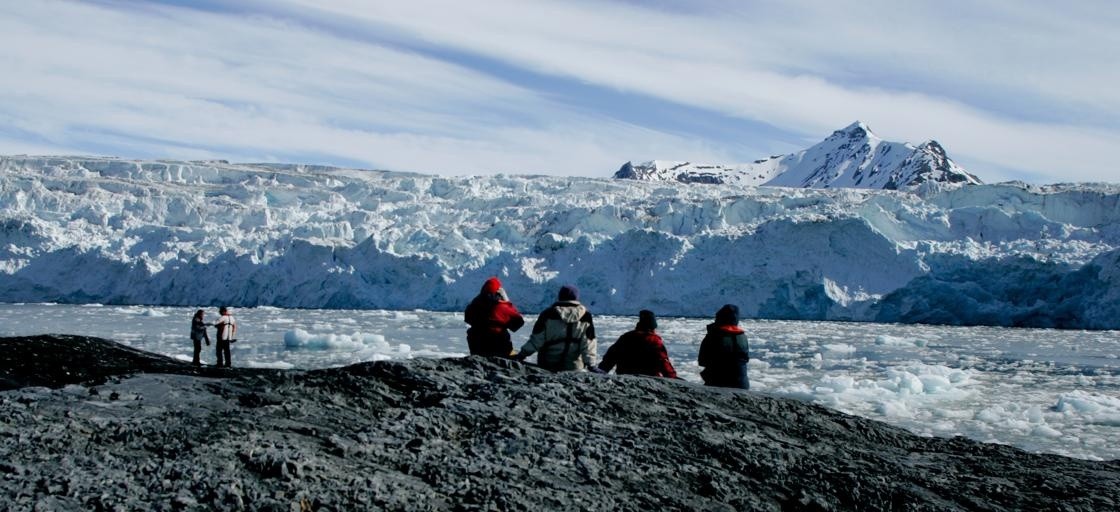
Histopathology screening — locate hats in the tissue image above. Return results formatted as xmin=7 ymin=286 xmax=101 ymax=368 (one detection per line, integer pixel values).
xmin=559 ymin=285 xmax=579 ymax=301
xmin=715 ymin=304 xmax=740 ymax=326
xmin=482 ymin=277 xmax=502 ymax=295
xmin=639 ymin=309 xmax=657 ymax=331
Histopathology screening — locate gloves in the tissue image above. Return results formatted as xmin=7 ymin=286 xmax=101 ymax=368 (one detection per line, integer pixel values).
xmin=513 ymin=352 xmax=525 ymax=363
xmin=496 ymin=288 xmax=510 ymax=302
xmin=588 ymin=365 xmax=606 ymax=374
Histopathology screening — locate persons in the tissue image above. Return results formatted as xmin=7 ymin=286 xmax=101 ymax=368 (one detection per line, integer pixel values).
xmin=697 ymin=304 xmax=751 ymax=390
xmin=189 ymin=310 xmax=211 ymax=363
xmin=464 ymin=278 xmax=525 ymax=358
xmin=511 ymin=283 xmax=605 ymax=374
xmin=212 ymin=306 xmax=236 ymax=368
xmin=597 ymin=310 xmax=686 ymax=381
